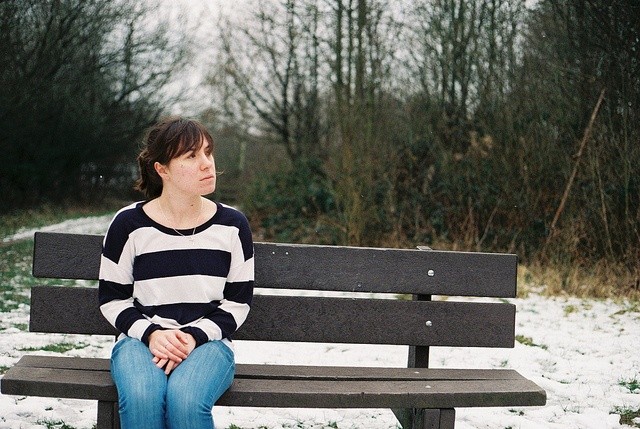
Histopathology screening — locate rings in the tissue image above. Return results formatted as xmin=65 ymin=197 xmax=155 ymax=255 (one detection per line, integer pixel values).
xmin=164 ymin=342 xmax=170 ymax=348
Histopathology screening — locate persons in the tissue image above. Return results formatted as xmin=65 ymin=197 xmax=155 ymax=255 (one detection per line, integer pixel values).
xmin=98 ymin=114 xmax=254 ymax=429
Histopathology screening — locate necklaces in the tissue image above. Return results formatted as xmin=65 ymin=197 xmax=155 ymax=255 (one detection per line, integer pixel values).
xmin=157 ymin=196 xmax=204 ymax=241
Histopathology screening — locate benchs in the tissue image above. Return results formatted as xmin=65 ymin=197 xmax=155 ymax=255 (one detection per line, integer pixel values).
xmin=0 ymin=231 xmax=546 ymax=429
